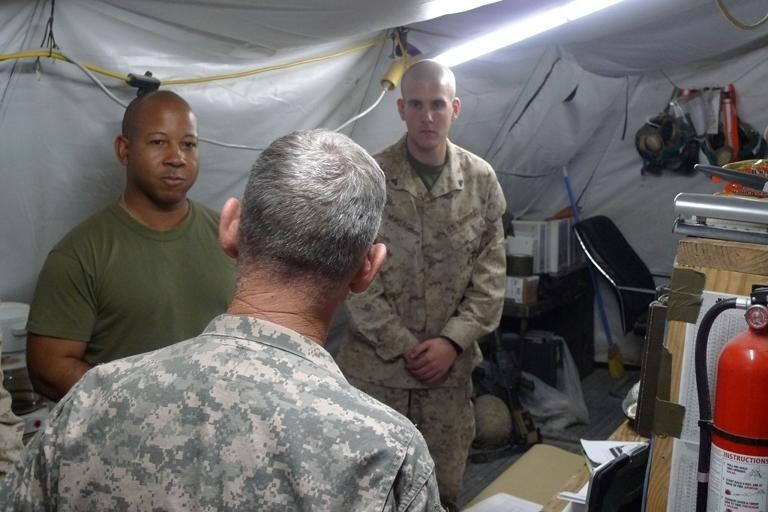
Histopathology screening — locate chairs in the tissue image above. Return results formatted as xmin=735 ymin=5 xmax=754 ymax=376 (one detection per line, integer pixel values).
xmin=569 ymin=216 xmax=669 ymax=336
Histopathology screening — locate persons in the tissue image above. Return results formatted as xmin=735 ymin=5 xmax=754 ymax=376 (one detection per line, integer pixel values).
xmin=333 ymin=57 xmax=511 ymax=512
xmin=0 ymin=124 xmax=450 ymax=512
xmin=25 ymin=89 xmax=240 ymax=405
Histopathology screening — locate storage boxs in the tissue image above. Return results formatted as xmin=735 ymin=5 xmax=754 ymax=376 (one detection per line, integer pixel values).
xmin=505 ymin=218 xmax=588 ymax=306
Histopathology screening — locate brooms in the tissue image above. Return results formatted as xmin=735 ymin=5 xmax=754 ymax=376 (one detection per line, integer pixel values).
xmin=561 ymin=163 xmax=626 ymax=383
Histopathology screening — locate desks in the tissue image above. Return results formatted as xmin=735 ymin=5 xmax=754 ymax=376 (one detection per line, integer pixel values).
xmin=488 ymin=281 xmax=589 ymax=445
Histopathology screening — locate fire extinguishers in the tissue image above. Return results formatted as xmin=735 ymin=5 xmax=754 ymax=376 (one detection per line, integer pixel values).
xmin=695 ymin=284 xmax=768 ymax=512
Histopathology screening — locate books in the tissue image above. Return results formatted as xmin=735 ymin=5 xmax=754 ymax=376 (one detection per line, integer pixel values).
xmin=580 ymin=435 xmax=649 ymax=474
